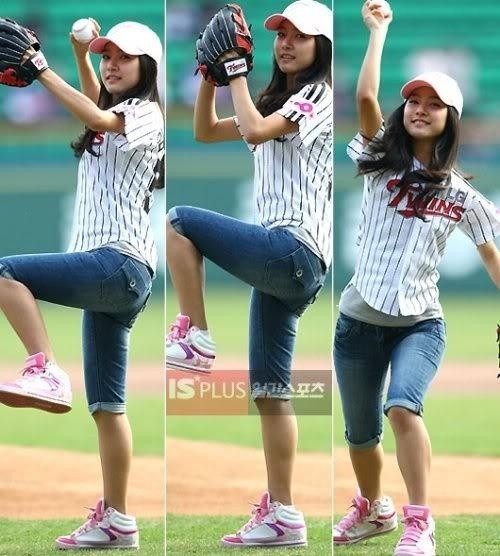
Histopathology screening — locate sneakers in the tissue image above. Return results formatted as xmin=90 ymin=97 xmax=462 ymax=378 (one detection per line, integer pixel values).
xmin=165 ymin=314 xmax=216 ymax=378
xmin=0 ymin=351 xmax=71 ymax=414
xmin=395 ymin=504 xmax=436 ymax=555
xmin=332 ymin=492 xmax=398 ymax=544
xmin=221 ymin=492 xmax=308 ymax=549
xmin=56 ymin=499 xmax=140 ymax=550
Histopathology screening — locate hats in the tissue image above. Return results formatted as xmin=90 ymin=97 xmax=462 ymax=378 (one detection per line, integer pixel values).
xmin=88 ymin=21 xmax=164 ymax=63
xmin=265 ymin=0 xmax=335 ymax=42
xmin=401 ymin=71 xmax=464 ymax=115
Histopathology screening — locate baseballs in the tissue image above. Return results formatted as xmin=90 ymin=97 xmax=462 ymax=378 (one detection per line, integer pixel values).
xmin=72 ymin=18 xmax=95 ymax=43
xmin=371 ymin=0 xmax=390 ymax=17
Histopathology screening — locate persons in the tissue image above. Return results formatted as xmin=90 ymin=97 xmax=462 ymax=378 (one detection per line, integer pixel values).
xmin=165 ymin=0 xmax=333 ymax=547
xmin=0 ymin=18 xmax=166 ymax=550
xmin=334 ymin=0 xmax=500 ymax=556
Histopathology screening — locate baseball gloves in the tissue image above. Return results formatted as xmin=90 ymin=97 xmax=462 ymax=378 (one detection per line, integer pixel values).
xmin=194 ymin=3 xmax=253 ymax=86
xmin=0 ymin=18 xmax=48 ymax=88
